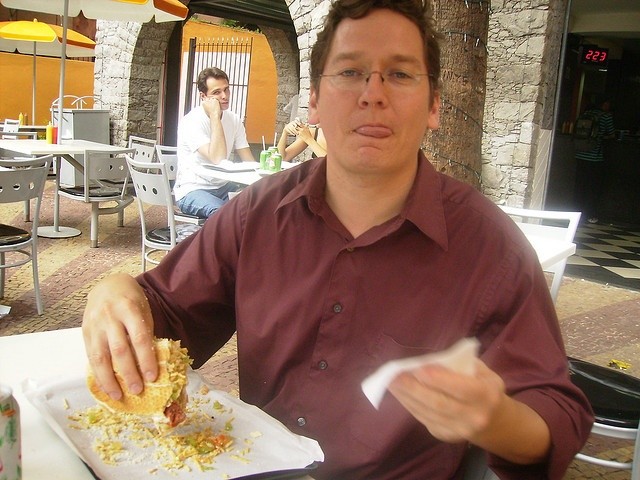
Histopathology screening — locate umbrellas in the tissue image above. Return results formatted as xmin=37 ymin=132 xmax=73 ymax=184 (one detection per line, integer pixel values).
xmin=1 ymin=18 xmax=97 ymax=126
xmin=1 ymin=1 xmax=188 ymax=239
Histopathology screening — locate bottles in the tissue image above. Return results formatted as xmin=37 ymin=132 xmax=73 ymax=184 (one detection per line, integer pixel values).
xmin=52 ymin=126 xmax=58 ymax=144
xmin=23 ymin=113 xmax=28 ymax=126
xmin=46 ymin=121 xmax=52 ymax=144
xmin=18 ymin=112 xmax=23 ymax=125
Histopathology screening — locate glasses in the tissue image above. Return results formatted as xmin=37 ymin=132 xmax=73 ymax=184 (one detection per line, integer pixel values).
xmin=318 ymin=66 xmax=431 ymax=85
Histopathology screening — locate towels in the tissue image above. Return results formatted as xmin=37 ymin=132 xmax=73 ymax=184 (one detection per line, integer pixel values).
xmin=202 ymin=163 xmax=255 ymax=173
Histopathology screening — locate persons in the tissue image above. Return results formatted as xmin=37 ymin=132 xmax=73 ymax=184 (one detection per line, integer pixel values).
xmin=573 ymin=94 xmax=616 ymax=224
xmin=277 ymin=119 xmax=328 ymax=162
xmin=83 ymin=1 xmax=595 ymax=480
xmin=173 ymin=67 xmax=257 ymax=220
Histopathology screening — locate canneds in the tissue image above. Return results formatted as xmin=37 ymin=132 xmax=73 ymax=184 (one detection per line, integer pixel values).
xmin=268 ymin=153 xmax=282 ymax=172
xmin=268 ymin=147 xmax=281 ymax=153
xmin=260 ymin=150 xmax=271 ymax=169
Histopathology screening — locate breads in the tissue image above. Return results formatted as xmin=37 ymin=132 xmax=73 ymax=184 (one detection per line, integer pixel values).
xmin=86 ymin=339 xmax=193 ymax=434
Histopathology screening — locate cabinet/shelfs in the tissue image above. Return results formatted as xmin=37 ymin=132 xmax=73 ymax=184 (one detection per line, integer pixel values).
xmin=50 ymin=108 xmax=112 ymax=188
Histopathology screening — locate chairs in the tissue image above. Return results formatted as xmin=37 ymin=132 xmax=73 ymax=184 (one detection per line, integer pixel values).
xmin=155 ymin=144 xmax=209 ymax=227
xmin=499 ymin=206 xmax=583 ymax=308
xmin=0 ymin=131 xmax=37 ymax=223
xmin=0 ymin=119 xmax=20 ymax=140
xmin=124 ymin=155 xmax=203 ymax=274
xmin=97 ymin=135 xmax=157 ymax=200
xmin=1 ymin=155 xmax=52 ymax=316
xmin=459 ymin=358 xmax=640 ymax=480
xmin=57 ymin=149 xmax=136 ymax=248
